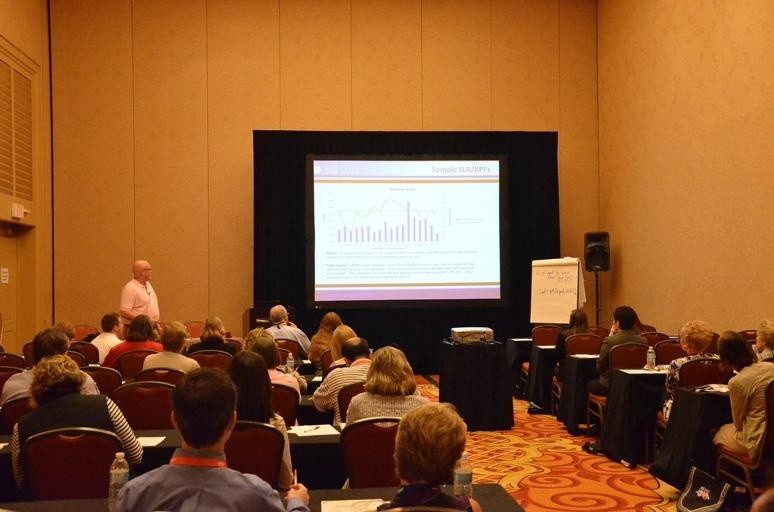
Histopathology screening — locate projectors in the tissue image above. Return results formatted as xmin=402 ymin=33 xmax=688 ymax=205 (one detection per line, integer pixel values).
xmin=451 ymin=327 xmax=494 ymax=344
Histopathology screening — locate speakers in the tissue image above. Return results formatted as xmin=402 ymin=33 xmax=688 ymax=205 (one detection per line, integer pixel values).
xmin=584 ymin=230 xmax=610 ymax=272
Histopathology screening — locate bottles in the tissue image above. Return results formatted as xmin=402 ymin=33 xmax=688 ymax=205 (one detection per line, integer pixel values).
xmin=452 ymin=452 xmax=474 ymax=498
xmin=106 ymin=451 xmax=131 ymax=512
xmin=646 ymin=346 xmax=656 ymax=369
xmin=286 ymin=351 xmax=294 ymax=371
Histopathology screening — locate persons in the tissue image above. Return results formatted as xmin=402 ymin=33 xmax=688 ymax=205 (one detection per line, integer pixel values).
xmin=2 ymin=313 xmax=163 ymax=495
xmin=584 ymin=306 xmax=648 ymax=435
xmin=607 ymin=307 xmax=655 ymax=337
xmin=659 ymin=320 xmax=720 ymax=423
xmin=190 ymin=303 xmax=431 ymax=487
xmin=757 ymin=320 xmax=774 ymax=362
xmin=142 ymin=321 xmax=202 ymax=374
xmin=711 ymin=330 xmax=774 ymax=460
xmin=552 ymin=309 xmax=593 ymax=382
xmin=120 ymin=260 xmax=162 ymax=340
xmin=112 ymin=367 xmax=311 ymax=511
xmin=373 ymin=402 xmax=481 ymax=512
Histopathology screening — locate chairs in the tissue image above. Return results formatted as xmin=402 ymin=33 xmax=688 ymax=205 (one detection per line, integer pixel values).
xmin=20 ymin=427 xmax=125 ymax=502
xmin=340 ymin=415 xmax=402 ymax=489
xmin=585 ymin=327 xmax=608 ymax=339
xmin=111 ymin=380 xmax=180 ymax=431
xmin=337 ymin=382 xmax=365 ymax=423
xmin=551 ymin=333 xmax=604 ymax=415
xmin=226 ymin=421 xmax=285 ymax=494
xmin=637 ymin=324 xmax=773 ymax=366
xmin=587 ymin=343 xmax=652 ymax=447
xmin=717 ymin=380 xmax=772 ymax=502
xmin=654 ymin=357 xmax=735 ymax=477
xmin=2 ymin=321 xmax=336 ymax=387
xmin=270 ymin=381 xmax=300 ymax=429
xmin=521 ymin=325 xmax=562 ymax=384
xmin=3 ymin=390 xmax=34 ymax=434
xmin=79 ymin=366 xmax=122 ymax=400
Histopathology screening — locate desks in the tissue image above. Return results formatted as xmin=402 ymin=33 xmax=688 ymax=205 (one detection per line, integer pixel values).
xmin=556 ymin=353 xmax=601 ymax=438
xmin=525 ymin=343 xmax=559 ymax=415
xmin=2 ymin=424 xmax=341 ymax=458
xmin=598 ymin=367 xmax=670 ymax=468
xmin=298 ymin=393 xmax=337 ymax=423
xmin=4 ymin=481 xmax=523 ymax=510
xmin=439 ymin=337 xmax=514 ymax=433
xmin=648 ymin=383 xmax=733 ymax=494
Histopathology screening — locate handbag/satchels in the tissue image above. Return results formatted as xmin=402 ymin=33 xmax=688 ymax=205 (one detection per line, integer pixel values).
xmin=676 ymin=465 xmax=732 ymax=512
